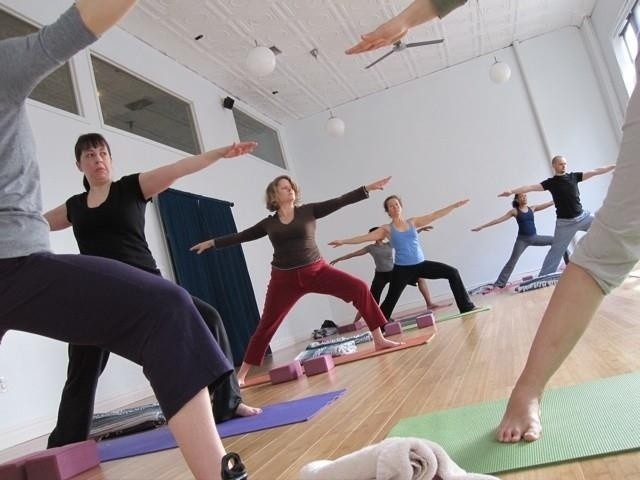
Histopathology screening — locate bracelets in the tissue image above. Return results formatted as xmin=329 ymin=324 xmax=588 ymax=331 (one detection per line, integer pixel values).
xmin=363 ymin=185 xmax=369 ymax=194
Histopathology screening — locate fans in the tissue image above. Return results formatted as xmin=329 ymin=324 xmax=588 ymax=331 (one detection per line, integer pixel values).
xmin=365 ymin=37 xmax=445 ymax=70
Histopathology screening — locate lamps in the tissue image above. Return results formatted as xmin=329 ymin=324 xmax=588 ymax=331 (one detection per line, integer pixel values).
xmin=476 ymin=0 xmax=511 ymax=84
xmin=244 ymin=0 xmax=277 ymax=78
xmin=326 ymin=112 xmax=344 ymax=138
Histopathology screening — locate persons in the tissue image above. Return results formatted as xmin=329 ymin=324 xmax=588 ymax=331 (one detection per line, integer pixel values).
xmin=327 ymin=195 xmax=482 ymax=315
xmin=344 ymin=0 xmax=640 ymax=445
xmin=471 ymin=193 xmax=571 ymax=290
xmin=497 ymin=155 xmax=616 ymax=277
xmin=42 ymin=133 xmax=262 ymax=452
xmin=329 ymin=225 xmax=447 ymax=324
xmin=188 ymin=174 xmax=407 ymax=388
xmin=0 ymin=1 xmax=236 ymax=479
